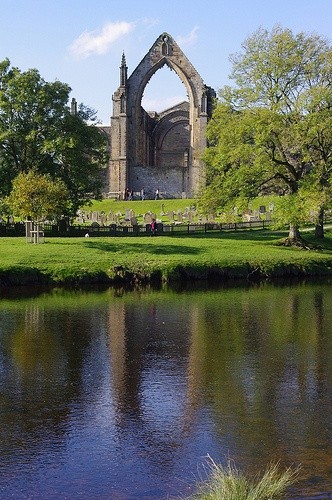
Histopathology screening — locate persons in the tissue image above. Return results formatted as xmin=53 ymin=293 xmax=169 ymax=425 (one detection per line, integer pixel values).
xmin=124 ymin=188 xmax=129 ymax=200
xmin=154 ymin=188 xmax=160 ymax=201
xmin=150 ymin=218 xmax=157 ymax=238
xmin=141 ymin=186 xmax=146 ymax=201
xmin=129 ymin=188 xmax=134 ymax=201
